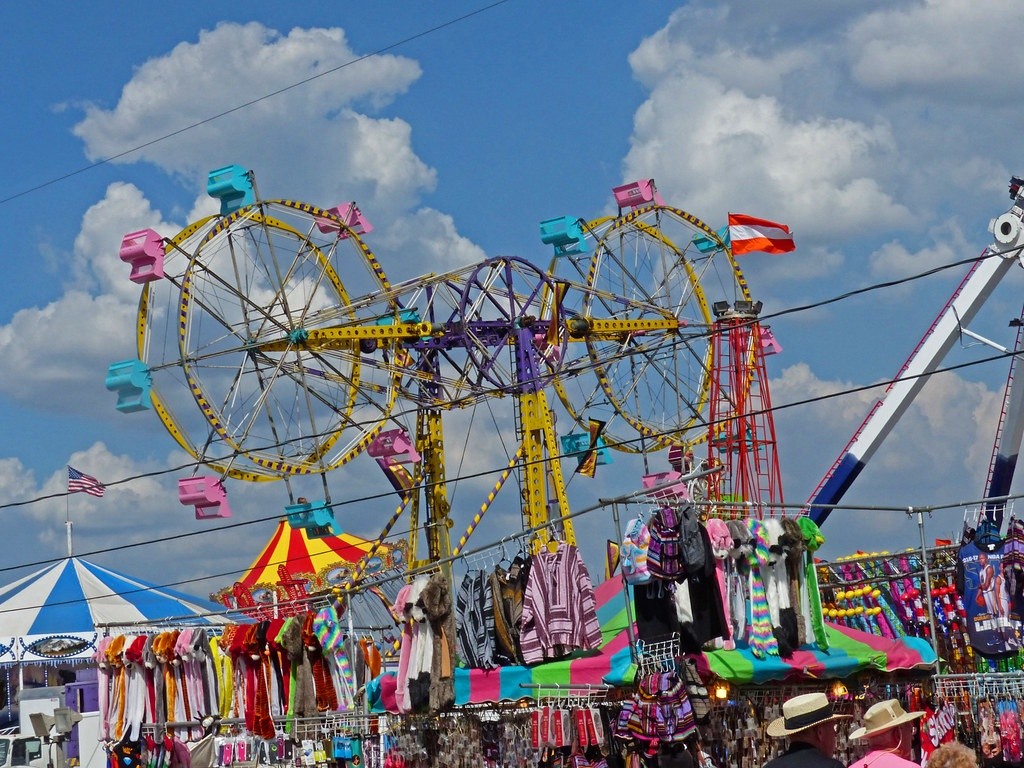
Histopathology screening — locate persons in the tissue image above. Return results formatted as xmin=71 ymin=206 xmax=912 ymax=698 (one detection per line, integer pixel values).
xmin=759 ymin=692 xmax=854 ymax=768
xmin=848 ymin=698 xmax=927 ymax=768
xmin=296 ymin=497 xmax=326 ymax=538
xmin=927 ymin=743 xmax=979 ymax=768
xmin=974 ymin=554 xmax=1010 ymax=619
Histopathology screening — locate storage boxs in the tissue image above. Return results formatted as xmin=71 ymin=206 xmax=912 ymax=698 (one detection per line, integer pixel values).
xmin=532 ymin=706 xmax=605 ymax=747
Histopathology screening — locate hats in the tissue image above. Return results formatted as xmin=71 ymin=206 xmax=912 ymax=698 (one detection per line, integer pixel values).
xmin=767 ymin=693 xmax=853 ymax=737
xmin=849 ymin=698 xmax=927 ymax=740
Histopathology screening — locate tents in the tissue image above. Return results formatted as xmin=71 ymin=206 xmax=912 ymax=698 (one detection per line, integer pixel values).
xmin=1 ymin=522 xmax=260 ymax=729
xmin=209 ymin=506 xmax=396 ymax=622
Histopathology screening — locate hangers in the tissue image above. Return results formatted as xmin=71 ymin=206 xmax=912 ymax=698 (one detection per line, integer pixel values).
xmin=461 ymin=520 xmax=561 ymax=579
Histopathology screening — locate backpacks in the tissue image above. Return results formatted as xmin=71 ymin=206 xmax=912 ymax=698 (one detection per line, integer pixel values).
xmin=618 ymin=498 xmax=704 ymax=599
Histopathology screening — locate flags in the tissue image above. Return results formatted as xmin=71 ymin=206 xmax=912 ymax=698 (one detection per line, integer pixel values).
xmin=66 ymin=465 xmax=107 ymax=498
xmin=728 ymin=210 xmax=796 ymax=259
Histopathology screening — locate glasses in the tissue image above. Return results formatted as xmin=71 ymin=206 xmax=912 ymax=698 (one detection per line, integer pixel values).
xmin=912 ymin=727 xmax=917 ymax=735
xmin=833 ymin=723 xmax=840 ymax=734
